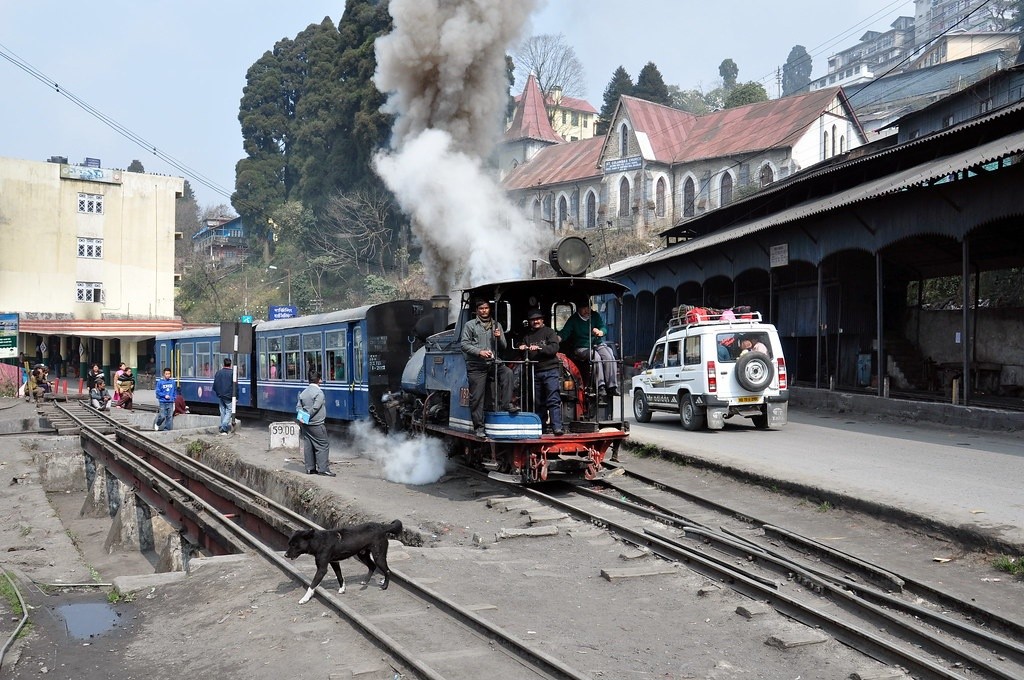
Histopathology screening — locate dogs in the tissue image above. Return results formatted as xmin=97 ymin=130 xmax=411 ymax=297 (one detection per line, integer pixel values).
xmin=284 ymin=519 xmax=403 ymax=605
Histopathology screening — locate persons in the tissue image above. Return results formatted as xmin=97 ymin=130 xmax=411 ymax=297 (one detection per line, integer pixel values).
xmin=94 ymin=377 xmax=101 ymax=387
xmin=91 ymin=379 xmax=112 ymax=411
xmin=212 ymin=358 xmax=239 ymax=434
xmin=19 ymin=351 xmax=25 ymax=367
xmin=71 ymin=349 xmax=80 ymax=378
xmin=154 ymin=368 xmax=177 ymax=431
xmin=746 ymin=333 xmax=770 ymax=358
xmin=461 ymin=299 xmax=521 ymax=438
xmin=203 ymin=363 xmax=209 ymax=376
xmin=557 ymin=295 xmax=621 ymax=396
xmin=288 ymin=363 xmax=300 ymax=379
xmin=717 ymin=334 xmax=731 ymax=361
xmin=145 ymin=356 xmax=156 ymax=390
xmin=295 ymin=370 xmax=336 ymax=477
xmin=51 ymin=349 xmax=63 ymax=379
xmin=173 ymin=386 xmax=190 ymax=414
xmin=24 ymin=363 xmax=55 ymax=403
xmin=334 ymin=356 xmax=344 ymax=380
xmin=330 ymin=351 xmax=334 ymax=380
xmin=270 ymin=359 xmax=278 ymax=379
xmin=516 ymin=308 xmax=566 ymax=434
xmin=736 ymin=339 xmax=753 ymax=359
xmin=115 ymin=367 xmax=135 ymax=410
xmin=112 ymin=362 xmax=126 ymax=401
xmin=87 ymin=363 xmax=105 ymax=400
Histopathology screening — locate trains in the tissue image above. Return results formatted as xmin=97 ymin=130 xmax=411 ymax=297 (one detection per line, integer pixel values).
xmin=156 ymin=237 xmax=631 ymax=485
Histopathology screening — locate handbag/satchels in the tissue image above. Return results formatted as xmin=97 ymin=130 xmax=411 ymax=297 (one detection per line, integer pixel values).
xmin=719 ymin=310 xmax=736 ymax=320
xmin=708 ymin=308 xmax=725 ymax=320
xmin=734 ymin=306 xmax=751 ymax=313
xmin=112 ymin=391 xmax=121 ymax=401
xmin=684 ymin=308 xmax=710 ymax=324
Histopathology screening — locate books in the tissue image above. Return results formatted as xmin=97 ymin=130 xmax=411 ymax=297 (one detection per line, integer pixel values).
xmin=297 ymin=410 xmax=310 ymax=425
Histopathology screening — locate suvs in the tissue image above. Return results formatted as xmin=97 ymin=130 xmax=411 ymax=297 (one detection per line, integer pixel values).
xmin=629 ymin=312 xmax=791 ymax=431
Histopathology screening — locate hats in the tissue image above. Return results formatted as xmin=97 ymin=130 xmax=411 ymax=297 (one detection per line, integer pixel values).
xmin=528 ymin=309 xmax=544 ymax=320
xmin=576 ymin=295 xmax=592 ymax=308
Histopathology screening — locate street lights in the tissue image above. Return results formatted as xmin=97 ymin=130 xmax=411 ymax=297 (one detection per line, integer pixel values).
xmin=269 ymin=265 xmax=291 ymax=307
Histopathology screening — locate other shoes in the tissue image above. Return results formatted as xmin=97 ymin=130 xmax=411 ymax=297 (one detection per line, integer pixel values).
xmin=505 ymin=402 xmax=521 ymax=413
xmin=598 ymin=385 xmax=607 ymax=396
xmin=553 ymin=429 xmax=565 ymax=434
xmin=97 ymin=407 xmax=104 ymax=410
xmin=106 ymin=407 xmax=110 ymax=411
xmin=307 ymin=470 xmax=318 ymax=474
xmin=116 ymin=406 xmax=121 ymax=409
xmin=318 ymin=471 xmax=336 ymax=476
xmin=219 ymin=431 xmax=229 ymax=436
xmin=477 ymin=430 xmax=486 ymax=437
xmin=607 ymin=387 xmax=621 ymax=395
xmin=155 ymin=424 xmax=159 ymax=431
